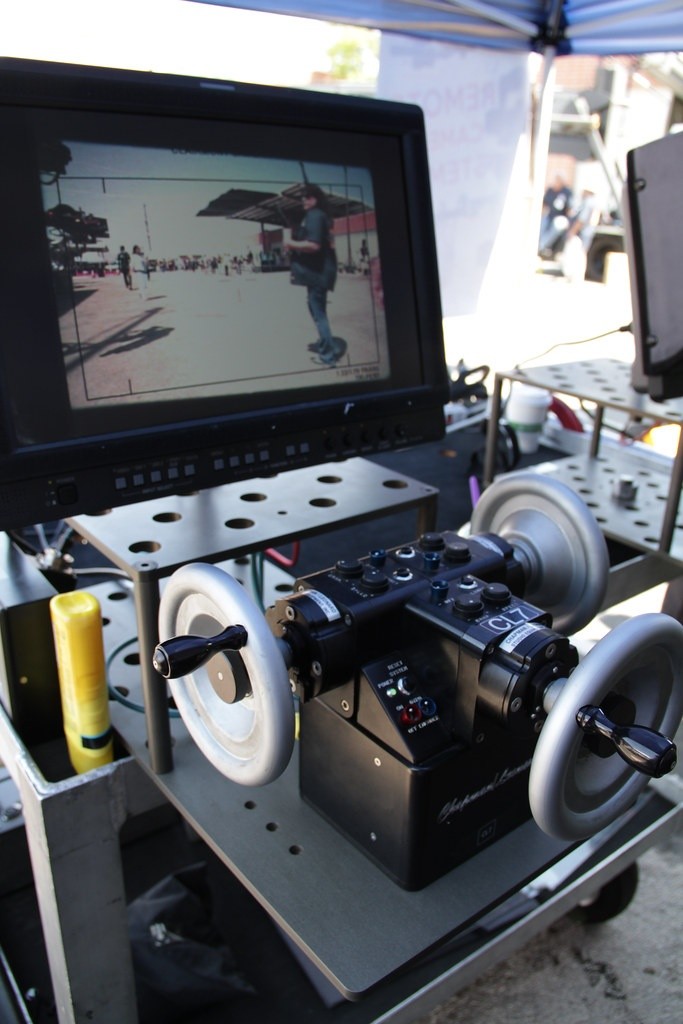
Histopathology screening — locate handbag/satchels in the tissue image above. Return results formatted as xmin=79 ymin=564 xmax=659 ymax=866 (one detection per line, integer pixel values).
xmin=289 ymin=249 xmax=336 ymax=290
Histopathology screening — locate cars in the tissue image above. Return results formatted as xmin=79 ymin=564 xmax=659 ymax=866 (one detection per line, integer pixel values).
xmin=74 ymin=260 xmax=158 ymax=276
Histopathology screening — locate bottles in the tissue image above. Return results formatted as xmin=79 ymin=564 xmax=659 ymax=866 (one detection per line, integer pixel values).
xmin=50 ymin=593 xmax=112 ymax=773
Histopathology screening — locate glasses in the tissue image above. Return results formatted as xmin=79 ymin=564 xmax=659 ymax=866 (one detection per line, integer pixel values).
xmin=300 ymin=194 xmax=312 ymax=198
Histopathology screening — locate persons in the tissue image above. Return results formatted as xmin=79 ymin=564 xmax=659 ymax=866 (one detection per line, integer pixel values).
xmin=93 ymin=245 xmax=368 ymax=291
xmin=285 ymin=184 xmax=337 ymax=366
xmin=536 ymin=171 xmax=599 ymax=265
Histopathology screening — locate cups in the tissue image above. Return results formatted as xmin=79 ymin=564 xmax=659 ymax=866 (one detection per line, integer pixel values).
xmin=503 ymin=383 xmax=552 ymax=454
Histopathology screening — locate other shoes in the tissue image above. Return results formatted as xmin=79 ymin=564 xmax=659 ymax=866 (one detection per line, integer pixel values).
xmin=306 ymin=337 xmax=326 ymax=355
xmin=311 ymin=356 xmax=334 ymax=367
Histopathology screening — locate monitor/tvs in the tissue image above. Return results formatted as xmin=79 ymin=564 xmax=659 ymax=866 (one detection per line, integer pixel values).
xmin=0 ymin=56 xmax=451 ymax=540
